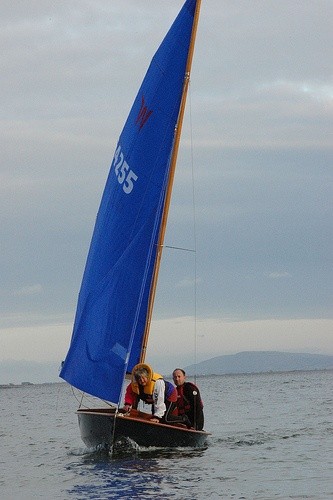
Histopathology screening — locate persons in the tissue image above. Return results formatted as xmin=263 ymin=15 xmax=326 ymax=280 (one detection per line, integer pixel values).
xmin=172 ymin=369 xmax=204 ymax=431
xmin=125 ymin=365 xmax=177 ymax=420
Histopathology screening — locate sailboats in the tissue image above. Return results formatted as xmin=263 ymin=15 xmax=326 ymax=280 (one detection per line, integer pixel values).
xmin=56 ymin=1 xmax=212 ymax=453
